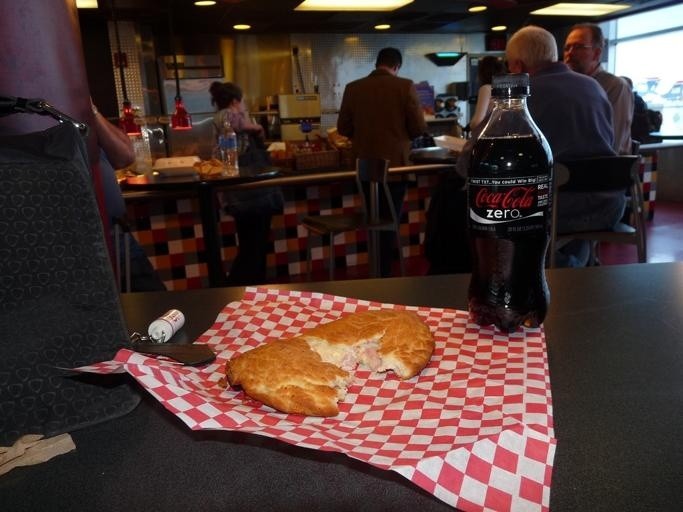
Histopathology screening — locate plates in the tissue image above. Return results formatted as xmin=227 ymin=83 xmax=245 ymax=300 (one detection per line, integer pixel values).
xmin=151 ymin=155 xmax=201 ymax=177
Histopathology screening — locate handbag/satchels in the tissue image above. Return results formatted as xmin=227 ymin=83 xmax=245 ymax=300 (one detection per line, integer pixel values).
xmin=0 ymin=94 xmax=142 ymax=445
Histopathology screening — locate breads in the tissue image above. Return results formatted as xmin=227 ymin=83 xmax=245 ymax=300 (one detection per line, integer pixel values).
xmin=225 ymin=307 xmax=435 ymax=417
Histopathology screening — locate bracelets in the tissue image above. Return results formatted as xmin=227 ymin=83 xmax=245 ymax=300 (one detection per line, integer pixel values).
xmin=93 ymin=110 xmax=99 ymax=115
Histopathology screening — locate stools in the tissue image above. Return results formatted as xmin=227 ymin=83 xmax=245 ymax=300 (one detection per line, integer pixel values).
xmin=548 ymin=141 xmax=647 ymax=268
xmin=294 ymin=157 xmax=400 ymax=280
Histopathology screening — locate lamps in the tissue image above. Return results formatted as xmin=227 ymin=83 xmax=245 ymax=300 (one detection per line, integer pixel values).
xmin=170 ymin=43 xmax=193 ymax=132
xmin=113 ymin=18 xmax=141 ymax=137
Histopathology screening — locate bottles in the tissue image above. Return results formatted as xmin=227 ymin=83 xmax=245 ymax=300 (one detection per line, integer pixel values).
xmin=466 ymin=73 xmax=558 ymax=332
xmin=217 ymin=117 xmax=241 ymax=177
xmin=117 ymin=106 xmax=157 ymax=177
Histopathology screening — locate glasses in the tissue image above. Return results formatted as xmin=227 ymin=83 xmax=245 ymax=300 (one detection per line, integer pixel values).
xmin=563 ymin=44 xmax=593 ymax=53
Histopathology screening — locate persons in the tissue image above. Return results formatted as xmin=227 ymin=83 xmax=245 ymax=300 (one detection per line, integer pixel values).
xmin=88 ymin=96 xmax=168 ymax=293
xmin=208 ymin=81 xmax=265 ymax=163
xmin=336 ymin=47 xmax=427 ymax=278
